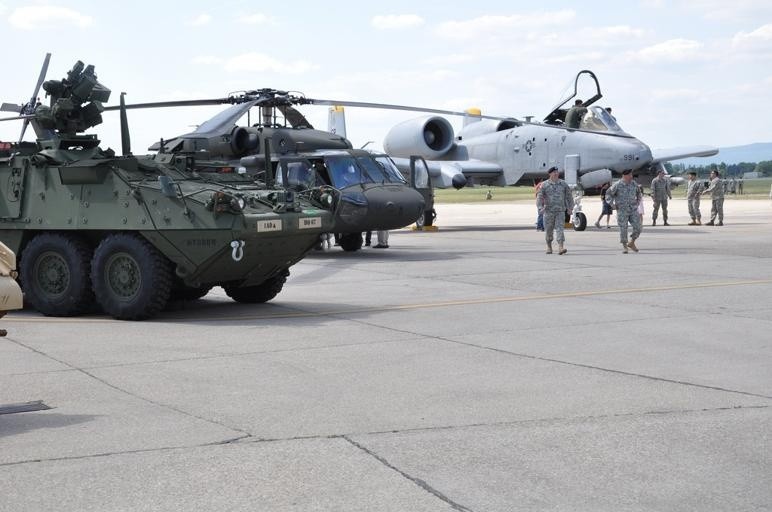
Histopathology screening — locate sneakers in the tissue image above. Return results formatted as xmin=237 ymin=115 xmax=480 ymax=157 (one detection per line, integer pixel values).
xmin=595 ymin=222 xmax=602 ymax=228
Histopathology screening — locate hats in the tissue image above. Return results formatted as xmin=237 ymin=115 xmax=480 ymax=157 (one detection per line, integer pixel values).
xmin=689 ymin=172 xmax=697 ymax=176
xmin=622 ymin=168 xmax=632 ymax=175
xmin=547 ymin=166 xmax=559 ymax=174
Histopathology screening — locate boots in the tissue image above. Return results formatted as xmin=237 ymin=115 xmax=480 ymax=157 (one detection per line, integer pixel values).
xmin=687 ymin=221 xmax=723 ymax=226
xmin=622 ymin=244 xmax=628 ymax=254
xmin=628 ymin=240 xmax=639 ymax=252
xmin=558 ymin=246 xmax=567 ymax=255
xmin=652 ymin=221 xmax=656 ymax=226
xmin=546 ymin=244 xmax=552 ymax=254
xmin=664 ymin=221 xmax=669 ymax=226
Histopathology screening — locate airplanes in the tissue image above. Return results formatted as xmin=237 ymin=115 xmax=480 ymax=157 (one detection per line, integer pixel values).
xmin=327 ymin=69 xmax=718 ymax=232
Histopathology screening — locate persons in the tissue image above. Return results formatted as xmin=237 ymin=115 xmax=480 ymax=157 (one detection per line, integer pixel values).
xmin=537 ymin=167 xmax=575 ymax=255
xmin=650 ymin=170 xmax=672 ymax=226
xmin=686 ymin=172 xmax=704 ymax=225
xmin=604 ymin=169 xmax=643 ymax=253
xmin=565 ymin=99 xmax=587 ymax=129
xmin=535 ymin=175 xmax=546 ymax=232
xmin=702 ymin=171 xmax=724 ymax=226
xmin=601 ymin=108 xmax=616 ymax=126
xmin=299 ymin=159 xmax=391 ymax=250
xmin=636 ymin=184 xmax=645 ymax=222
xmin=721 ymin=176 xmax=745 ymax=194
xmin=595 ymin=182 xmax=613 ymax=228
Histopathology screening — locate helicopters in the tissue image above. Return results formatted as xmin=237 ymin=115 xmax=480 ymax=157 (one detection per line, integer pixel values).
xmin=1 ymin=54 xmax=634 ymax=252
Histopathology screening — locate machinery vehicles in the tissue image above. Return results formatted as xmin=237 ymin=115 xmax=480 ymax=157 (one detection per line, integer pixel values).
xmin=1 ymin=60 xmax=336 ymax=321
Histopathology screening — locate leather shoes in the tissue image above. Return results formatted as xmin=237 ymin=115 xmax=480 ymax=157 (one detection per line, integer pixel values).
xmin=365 ymin=243 xmax=389 ymax=248
